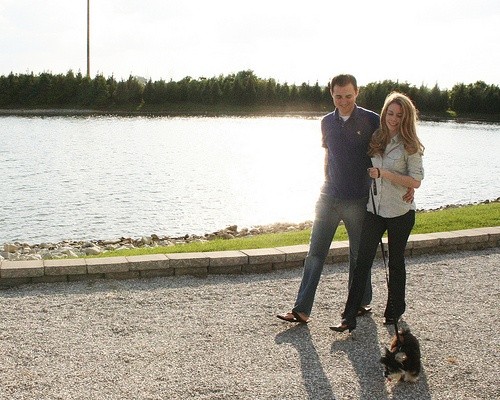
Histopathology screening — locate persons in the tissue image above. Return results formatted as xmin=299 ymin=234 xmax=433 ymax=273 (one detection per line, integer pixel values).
xmin=275 ymin=74 xmax=415 ymax=323
xmin=328 ymin=92 xmax=424 ymax=333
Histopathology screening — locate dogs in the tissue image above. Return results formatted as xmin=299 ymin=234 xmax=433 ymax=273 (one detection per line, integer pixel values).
xmin=379 ymin=328 xmax=421 ymax=382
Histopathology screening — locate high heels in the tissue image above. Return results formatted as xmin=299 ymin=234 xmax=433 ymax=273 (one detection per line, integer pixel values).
xmin=330 ymin=322 xmax=360 ymax=333
xmin=382 ymin=315 xmax=401 ymax=324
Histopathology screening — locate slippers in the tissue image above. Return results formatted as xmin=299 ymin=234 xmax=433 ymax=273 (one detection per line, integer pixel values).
xmin=276 ymin=309 xmax=311 ymax=324
xmin=341 ymin=304 xmax=373 ymax=319
